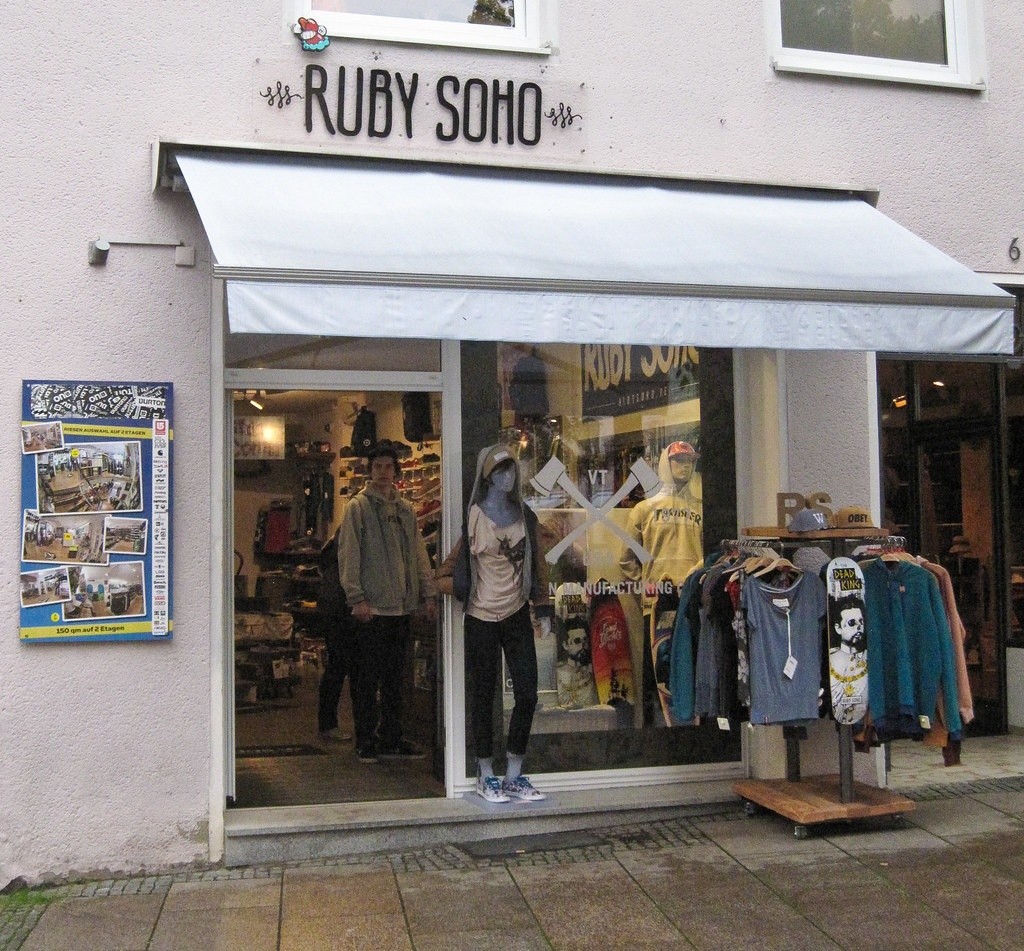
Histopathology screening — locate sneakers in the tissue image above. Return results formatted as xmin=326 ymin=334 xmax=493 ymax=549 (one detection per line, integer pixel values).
xmin=477 ymin=774 xmax=510 ymax=803
xmin=502 ymin=776 xmax=546 ymax=801
xmin=341 ymin=452 xmax=440 ymax=568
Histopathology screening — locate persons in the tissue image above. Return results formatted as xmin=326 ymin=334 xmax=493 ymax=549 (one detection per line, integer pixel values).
xmin=434 ymin=441 xmax=546 ymax=802
xmin=336 ymin=439 xmax=438 ymax=762
xmin=619 ymin=440 xmax=703 ymax=729
xmin=313 ymin=525 xmax=361 ymax=755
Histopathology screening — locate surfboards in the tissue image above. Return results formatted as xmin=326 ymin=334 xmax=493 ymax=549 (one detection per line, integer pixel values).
xmin=589 ymin=577 xmax=636 ymax=706
xmin=649 ymin=572 xmax=701 ymax=728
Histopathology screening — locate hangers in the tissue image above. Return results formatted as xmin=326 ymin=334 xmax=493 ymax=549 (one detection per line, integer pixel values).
xmin=687 ymin=536 xmax=928 ymax=592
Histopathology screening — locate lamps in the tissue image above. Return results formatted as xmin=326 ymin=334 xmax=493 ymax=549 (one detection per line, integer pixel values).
xmin=932 ymin=378 xmax=945 ymax=386
xmin=892 ymin=395 xmax=908 ymax=408
xmin=250 ymin=390 xmax=266 ymax=410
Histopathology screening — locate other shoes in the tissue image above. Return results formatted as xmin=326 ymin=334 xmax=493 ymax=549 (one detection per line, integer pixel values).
xmin=379 ymin=741 xmax=428 ymax=760
xmin=356 ymin=742 xmax=378 ymax=763
xmin=320 ymin=728 xmax=352 ymax=740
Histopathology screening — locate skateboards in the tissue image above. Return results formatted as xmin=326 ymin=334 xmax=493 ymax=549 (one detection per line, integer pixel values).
xmin=555 ymin=582 xmax=594 ymax=708
xmin=825 ymin=556 xmax=869 ymax=726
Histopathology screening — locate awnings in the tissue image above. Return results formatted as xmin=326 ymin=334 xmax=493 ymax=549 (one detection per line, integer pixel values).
xmin=172 ymin=145 xmax=1013 ymax=355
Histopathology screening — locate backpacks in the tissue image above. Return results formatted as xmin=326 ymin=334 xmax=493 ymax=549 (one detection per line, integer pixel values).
xmin=402 ymin=391 xmax=433 ymax=452
xmin=351 ymin=406 xmax=377 ymax=458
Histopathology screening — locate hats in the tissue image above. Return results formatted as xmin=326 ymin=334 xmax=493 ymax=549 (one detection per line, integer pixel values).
xmin=787 ymin=509 xmax=837 ymax=533
xmin=668 ymin=441 xmax=701 ymax=460
xmin=832 ymin=506 xmax=879 ymax=528
xmin=483 ymin=446 xmax=518 ymax=478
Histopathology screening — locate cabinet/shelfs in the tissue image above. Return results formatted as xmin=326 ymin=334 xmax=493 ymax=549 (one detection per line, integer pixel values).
xmin=252 ymin=448 xmax=337 ymax=674
xmin=731 ymin=527 xmax=917 ymax=840
xmin=337 ymin=436 xmax=442 ymax=577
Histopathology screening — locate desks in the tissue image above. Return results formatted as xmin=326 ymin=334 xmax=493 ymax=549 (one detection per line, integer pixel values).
xmin=234 ymin=610 xmax=300 ymax=703
